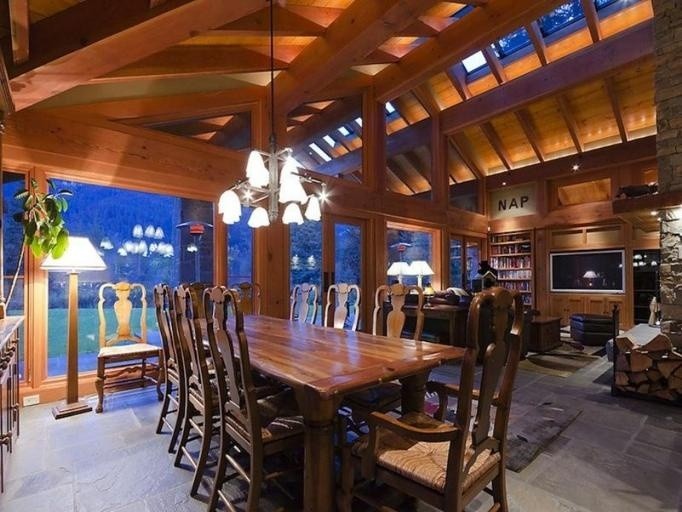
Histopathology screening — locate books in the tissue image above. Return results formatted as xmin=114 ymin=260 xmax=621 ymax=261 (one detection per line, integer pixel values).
xmin=491 ymin=235 xmax=532 ymax=304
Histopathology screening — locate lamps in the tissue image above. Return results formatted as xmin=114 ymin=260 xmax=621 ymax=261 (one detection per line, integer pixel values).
xmin=39 ymin=236 xmax=108 ymax=419
xmin=217 ymin=0 xmax=323 ymax=230
xmin=387 ymin=260 xmax=434 ymax=292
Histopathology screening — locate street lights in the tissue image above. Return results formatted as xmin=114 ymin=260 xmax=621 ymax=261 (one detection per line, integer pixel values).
xmin=100 ymin=224 xmax=174 ymax=276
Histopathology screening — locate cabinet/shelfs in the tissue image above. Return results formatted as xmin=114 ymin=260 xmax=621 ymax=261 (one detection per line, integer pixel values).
xmin=526 ymin=316 xmax=563 ymax=353
xmin=489 ymin=231 xmax=535 ymax=314
xmin=0 ymin=326 xmax=19 ymax=493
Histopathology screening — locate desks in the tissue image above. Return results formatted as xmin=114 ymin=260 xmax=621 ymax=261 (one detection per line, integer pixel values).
xmin=189 ymin=315 xmax=467 ymax=511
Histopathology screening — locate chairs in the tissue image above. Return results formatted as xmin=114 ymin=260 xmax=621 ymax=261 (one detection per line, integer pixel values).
xmin=324 ymin=284 xmax=362 ymax=331
xmin=153 ymin=283 xmax=346 ymax=511
xmin=341 ymin=287 xmax=525 ymax=512
xmin=95 ymin=281 xmax=164 ymax=414
xmin=372 ymin=284 xmax=424 ymax=341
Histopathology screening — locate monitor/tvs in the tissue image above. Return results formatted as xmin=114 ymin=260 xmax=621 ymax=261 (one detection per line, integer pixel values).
xmin=549 ymin=249 xmax=626 ymax=294
